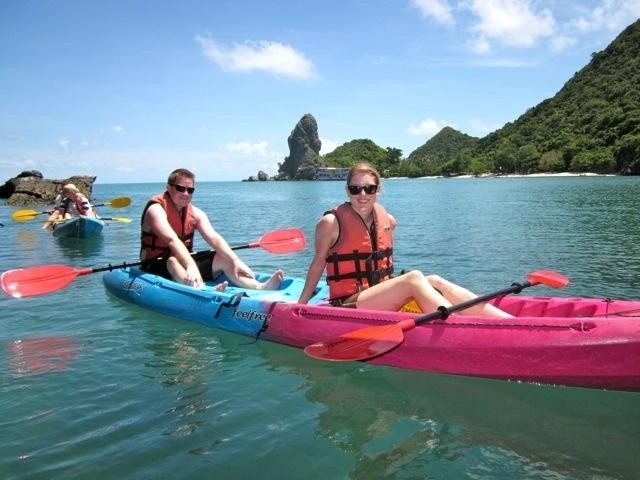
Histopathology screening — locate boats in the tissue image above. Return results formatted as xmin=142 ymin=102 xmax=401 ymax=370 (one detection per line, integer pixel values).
xmin=102 ymin=260 xmax=640 ymax=392
xmin=51 ymin=209 xmax=105 ymax=238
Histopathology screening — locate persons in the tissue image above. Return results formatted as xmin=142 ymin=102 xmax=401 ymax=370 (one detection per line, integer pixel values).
xmin=296 ymin=162 xmax=517 ymax=320
xmin=139 ymin=168 xmax=284 ymax=295
xmin=42 ymin=179 xmax=99 ymax=231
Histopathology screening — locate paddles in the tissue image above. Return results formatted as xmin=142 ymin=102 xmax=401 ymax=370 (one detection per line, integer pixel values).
xmin=304 ymin=270 xmax=570 ymax=362
xmin=39 ymin=217 xmax=131 ymax=224
xmin=12 ymin=197 xmax=130 ymax=222
xmin=0 ymin=228 xmax=306 ymax=298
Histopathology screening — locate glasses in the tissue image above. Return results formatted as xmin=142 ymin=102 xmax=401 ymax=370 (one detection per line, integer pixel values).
xmin=170 ymin=183 xmax=194 ymax=194
xmin=347 ymin=184 xmax=377 ymax=195
